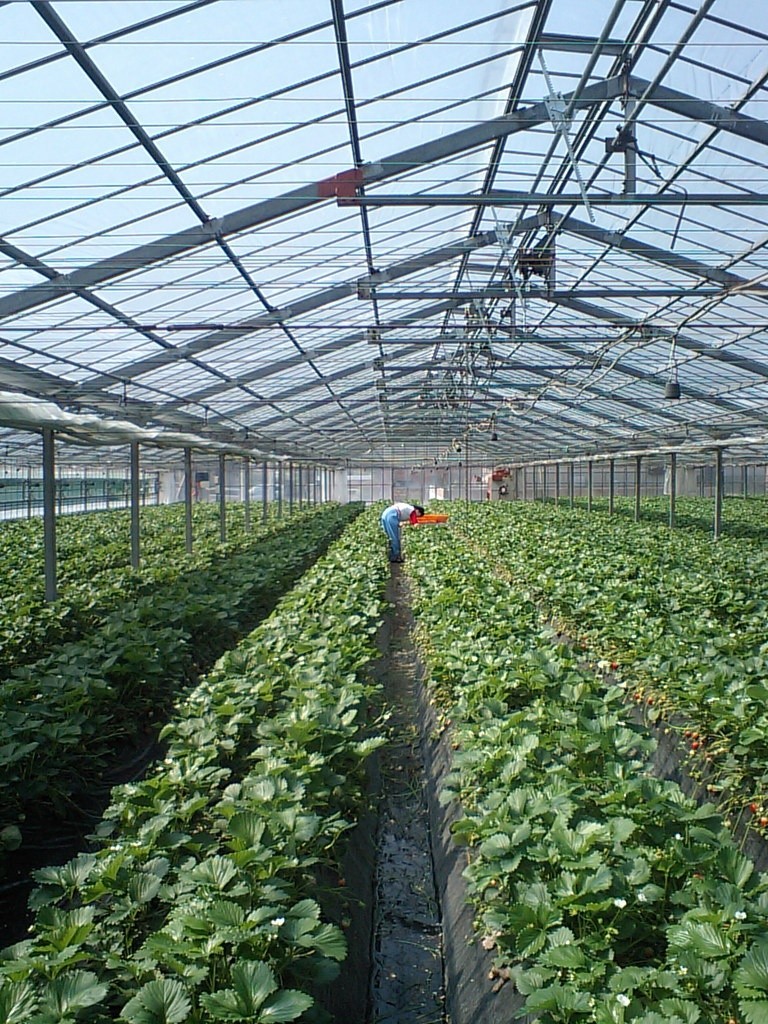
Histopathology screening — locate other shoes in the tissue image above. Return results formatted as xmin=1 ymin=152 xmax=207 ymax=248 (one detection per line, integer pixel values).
xmin=391 ymin=558 xmax=404 ymax=563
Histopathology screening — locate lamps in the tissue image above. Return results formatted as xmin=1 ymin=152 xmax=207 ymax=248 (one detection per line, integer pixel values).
xmin=664 ymin=338 xmax=681 ymax=403
xmin=430 ymin=444 xmax=463 ymax=473
xmin=492 ymin=421 xmax=498 ymax=442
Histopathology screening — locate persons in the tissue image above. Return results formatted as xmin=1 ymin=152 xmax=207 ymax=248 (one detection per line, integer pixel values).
xmin=215 ymin=483 xmax=221 ymax=502
xmin=381 ymin=503 xmax=425 ymax=564
